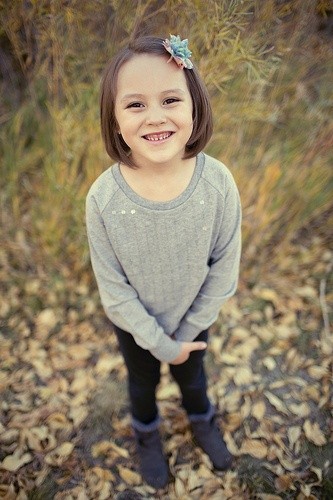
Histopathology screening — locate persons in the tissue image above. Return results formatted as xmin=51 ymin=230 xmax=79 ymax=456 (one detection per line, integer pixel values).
xmin=84 ymin=36 xmax=245 ymax=490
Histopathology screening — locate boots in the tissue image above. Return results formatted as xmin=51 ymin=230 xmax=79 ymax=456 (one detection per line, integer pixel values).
xmin=131 ymin=405 xmax=170 ymax=489
xmin=187 ymin=403 xmax=233 ymax=469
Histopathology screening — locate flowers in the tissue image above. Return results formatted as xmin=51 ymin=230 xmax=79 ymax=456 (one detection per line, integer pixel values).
xmin=161 ymin=31 xmax=195 ymax=72
